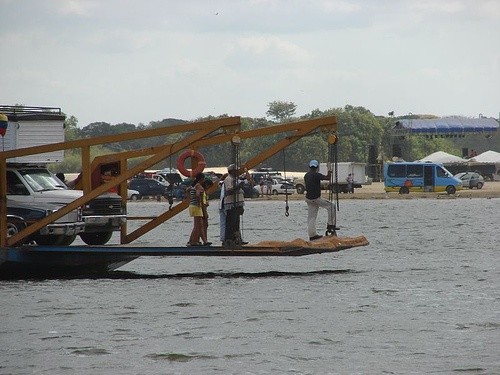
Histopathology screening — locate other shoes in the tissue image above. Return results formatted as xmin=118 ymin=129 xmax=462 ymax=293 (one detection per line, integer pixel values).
xmin=204 ymin=240 xmax=212 ymax=245
xmin=190 ymin=242 xmax=204 ymax=246
xmin=239 ymin=240 xmax=248 ymax=244
xmin=310 ymin=234 xmax=323 ymax=240
xmin=327 ymin=225 xmax=341 ymax=230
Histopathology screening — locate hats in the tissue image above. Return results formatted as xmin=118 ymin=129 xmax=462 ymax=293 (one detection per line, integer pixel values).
xmin=228 ymin=164 xmax=237 ymax=171
xmin=309 ymin=159 xmax=318 ymax=167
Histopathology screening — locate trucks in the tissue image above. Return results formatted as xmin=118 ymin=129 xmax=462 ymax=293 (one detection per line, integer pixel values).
xmin=293 ymin=161 xmax=366 ymax=194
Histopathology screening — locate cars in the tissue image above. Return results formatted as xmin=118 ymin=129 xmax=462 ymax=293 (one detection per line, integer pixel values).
xmin=127 ymin=171 xmax=295 ymax=201
xmin=454 ymin=172 xmax=484 ymax=189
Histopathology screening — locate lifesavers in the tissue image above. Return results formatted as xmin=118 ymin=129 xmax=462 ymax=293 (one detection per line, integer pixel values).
xmin=176 ymin=149 xmax=206 ymax=178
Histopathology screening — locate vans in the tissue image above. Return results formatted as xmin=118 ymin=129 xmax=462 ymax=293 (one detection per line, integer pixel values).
xmin=384 ymin=161 xmax=461 ymax=194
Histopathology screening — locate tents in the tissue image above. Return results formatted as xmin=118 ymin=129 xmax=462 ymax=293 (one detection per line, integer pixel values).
xmin=418 ymin=150 xmax=467 ymax=171
xmin=464 ymin=149 xmax=500 ymax=172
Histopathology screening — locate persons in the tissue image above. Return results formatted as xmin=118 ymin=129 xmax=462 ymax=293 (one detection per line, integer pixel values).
xmin=304 ymin=159 xmax=341 ymax=241
xmin=218 ymin=163 xmax=248 ymax=246
xmin=186 ymin=172 xmax=213 ymax=246
xmin=266 ymin=176 xmax=272 ymax=197
xmin=473 ymin=152 xmax=477 ymax=156
xmin=259 ymin=176 xmax=264 ymax=198
xmin=475 ymin=168 xmax=492 ymax=181
xmin=470 ymin=149 xmax=474 ymax=157
xmin=345 ymin=174 xmax=354 ymax=194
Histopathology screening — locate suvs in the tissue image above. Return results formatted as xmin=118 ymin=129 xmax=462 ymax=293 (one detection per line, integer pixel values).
xmin=6 ymin=167 xmax=126 ymax=246
